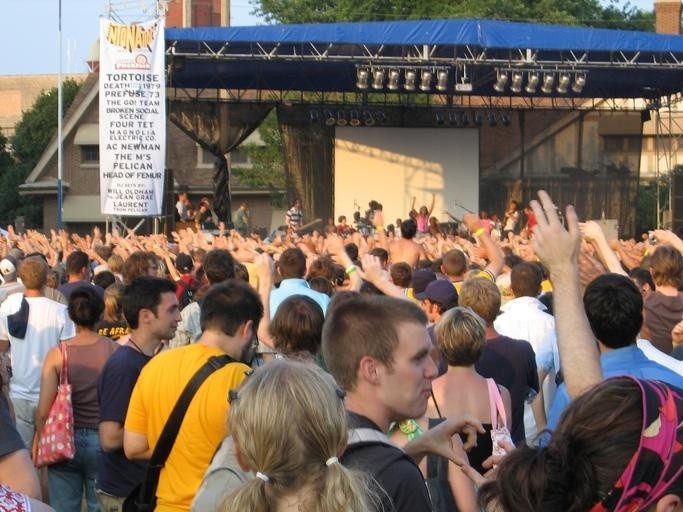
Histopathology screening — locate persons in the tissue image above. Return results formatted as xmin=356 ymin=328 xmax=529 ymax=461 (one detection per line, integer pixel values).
xmin=1 ymin=191 xmax=683 ymax=511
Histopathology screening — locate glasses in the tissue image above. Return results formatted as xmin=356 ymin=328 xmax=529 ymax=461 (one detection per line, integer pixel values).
xmin=254 ymin=330 xmax=260 ymax=351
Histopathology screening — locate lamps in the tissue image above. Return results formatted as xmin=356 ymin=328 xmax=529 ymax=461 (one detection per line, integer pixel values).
xmin=430 ymin=105 xmax=513 ymax=129
xmin=307 ymin=103 xmax=389 ymax=128
xmin=492 ymin=58 xmax=588 ymax=94
xmin=353 ymin=57 xmax=452 ymax=92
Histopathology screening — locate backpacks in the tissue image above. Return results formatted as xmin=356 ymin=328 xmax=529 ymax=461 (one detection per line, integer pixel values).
xmin=178 ymin=279 xmax=194 ymax=307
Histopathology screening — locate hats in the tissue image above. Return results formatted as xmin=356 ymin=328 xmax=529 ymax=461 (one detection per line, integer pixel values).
xmin=1 ymin=256 xmax=17 ymax=275
xmin=414 ymin=280 xmax=458 ymax=305
xmin=176 ymin=252 xmax=193 ymax=271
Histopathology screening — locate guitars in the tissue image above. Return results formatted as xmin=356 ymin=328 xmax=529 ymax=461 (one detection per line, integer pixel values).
xmin=501 ymin=210 xmax=514 ymax=232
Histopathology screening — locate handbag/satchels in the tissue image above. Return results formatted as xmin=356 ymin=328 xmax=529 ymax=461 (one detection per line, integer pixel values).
xmin=486 ymin=378 xmax=515 ymax=469
xmin=35 ymin=340 xmax=76 ymax=468
xmin=122 ymin=355 xmax=233 ymax=512
